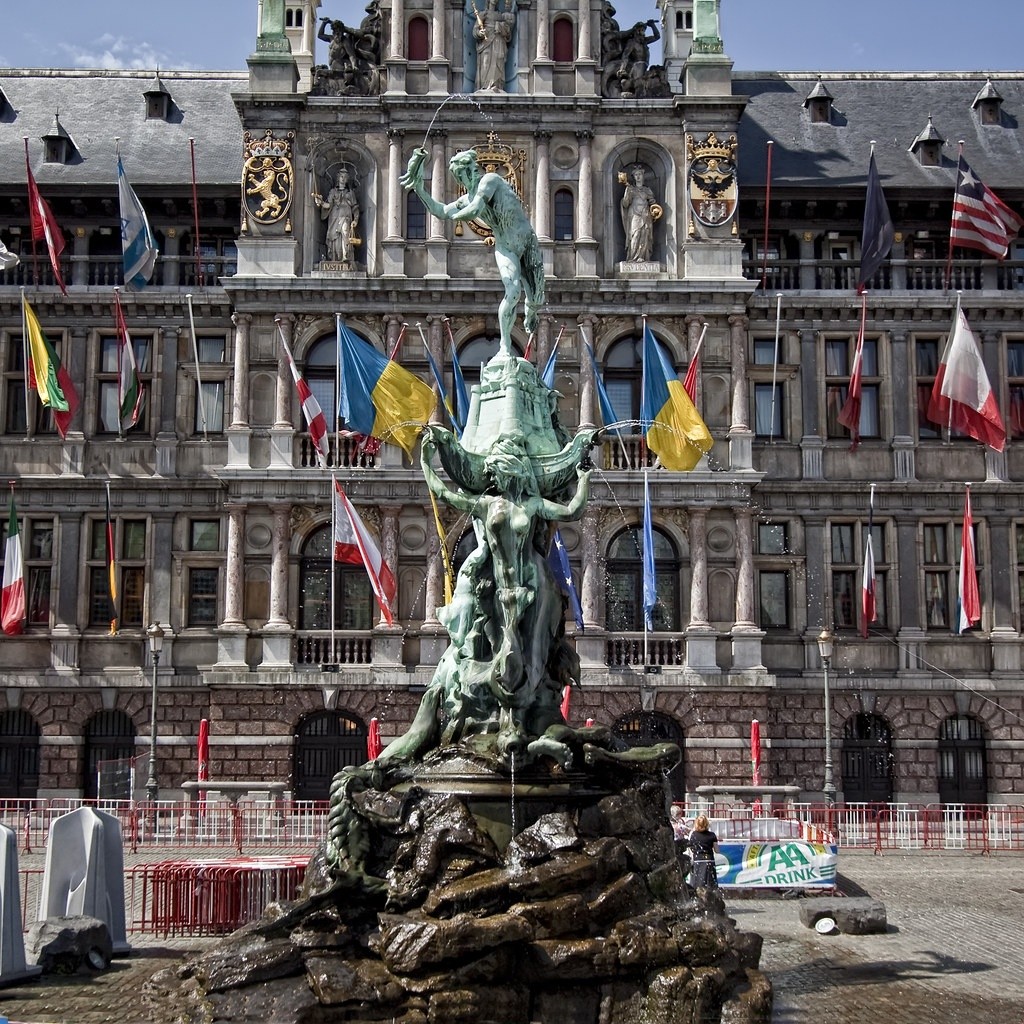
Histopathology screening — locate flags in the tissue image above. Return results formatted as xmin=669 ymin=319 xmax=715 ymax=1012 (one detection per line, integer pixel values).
xmin=0 ymin=153 xmax=155 ymax=634
xmin=526 ymin=336 xmax=622 ymax=433
xmin=549 ymin=528 xmax=585 ymax=630
xmin=643 ymin=475 xmax=657 ymax=633
xmin=288 ymin=323 xmax=469 ymax=627
xmin=835 ymin=311 xmax=1008 ymax=631
xmin=950 ymin=155 xmax=1021 ymax=261
xmin=641 ymin=325 xmax=714 ymax=470
xmin=857 ymin=154 xmax=894 ymax=295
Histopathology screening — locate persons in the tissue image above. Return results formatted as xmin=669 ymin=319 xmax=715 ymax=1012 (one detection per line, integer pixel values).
xmin=618 ymin=20 xmax=660 ymax=96
xmin=671 ymin=807 xmax=719 ymax=888
xmin=412 ymin=149 xmax=545 ymax=361
xmin=419 ymin=431 xmax=683 ymax=778
xmin=473 ymin=0 xmax=512 ymax=90
xmin=317 ymin=16 xmax=359 ymax=85
xmin=620 ymin=165 xmax=661 ymax=263
xmin=315 ymin=169 xmax=360 ymax=260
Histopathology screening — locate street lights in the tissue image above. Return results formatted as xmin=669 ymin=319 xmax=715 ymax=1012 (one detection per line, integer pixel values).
xmin=815 ymin=626 xmax=838 ymax=838
xmin=144 ymin=621 xmax=166 ymax=835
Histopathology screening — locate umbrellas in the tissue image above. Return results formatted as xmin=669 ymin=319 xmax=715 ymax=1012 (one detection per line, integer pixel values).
xmin=198 ymin=720 xmax=208 ymax=841
xmin=368 ymin=718 xmax=381 ymax=761
xmin=751 ymin=721 xmax=762 ymax=815
